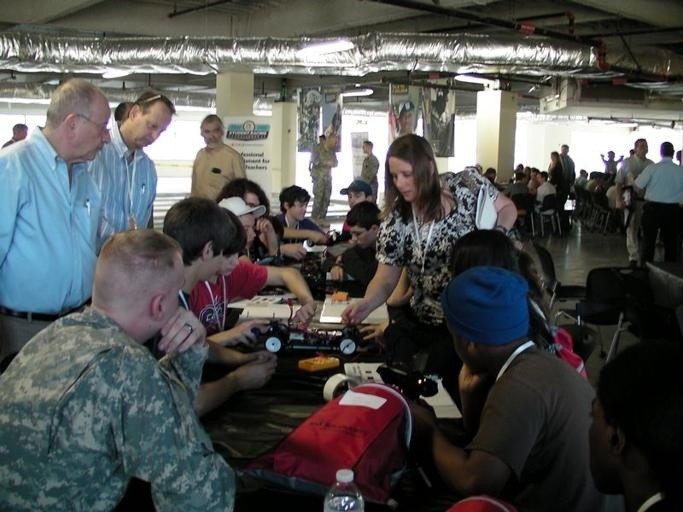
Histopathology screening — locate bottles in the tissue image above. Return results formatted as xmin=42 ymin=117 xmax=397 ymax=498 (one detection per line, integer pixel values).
xmin=323 ymin=468 xmax=364 ymax=512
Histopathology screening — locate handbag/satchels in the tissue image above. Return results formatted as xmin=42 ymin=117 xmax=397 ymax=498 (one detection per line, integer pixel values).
xmin=616 ymin=185 xmax=633 ymax=208
xmin=237 ymin=382 xmax=416 ymax=506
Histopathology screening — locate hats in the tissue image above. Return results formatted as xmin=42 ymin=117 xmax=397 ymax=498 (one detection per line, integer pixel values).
xmin=441 ymin=266 xmax=531 ymax=346
xmin=216 ymin=197 xmax=267 ymax=220
xmin=340 ymin=181 xmax=372 ymax=196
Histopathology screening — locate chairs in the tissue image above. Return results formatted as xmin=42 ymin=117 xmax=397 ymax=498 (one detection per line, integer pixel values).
xmin=509 ymin=182 xmax=623 ymax=244
xmin=604 ymin=257 xmax=683 ymax=363
xmin=550 ymin=261 xmax=639 ymax=363
xmin=532 ymin=237 xmax=586 ymax=322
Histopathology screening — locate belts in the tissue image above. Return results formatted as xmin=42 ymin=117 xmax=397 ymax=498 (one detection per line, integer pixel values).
xmin=1 ymin=296 xmax=92 ymax=323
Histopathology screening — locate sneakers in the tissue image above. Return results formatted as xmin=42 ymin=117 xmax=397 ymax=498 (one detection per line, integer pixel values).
xmin=312 ymin=218 xmax=331 ymax=227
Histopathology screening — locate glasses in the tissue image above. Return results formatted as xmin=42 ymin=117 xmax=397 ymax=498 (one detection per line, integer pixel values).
xmin=61 ymin=113 xmax=110 ymax=137
xmin=139 ymin=95 xmax=177 ymax=113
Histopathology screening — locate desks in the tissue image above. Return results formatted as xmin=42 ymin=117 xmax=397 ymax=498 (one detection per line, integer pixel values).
xmin=121 ymin=253 xmax=485 ymax=509
